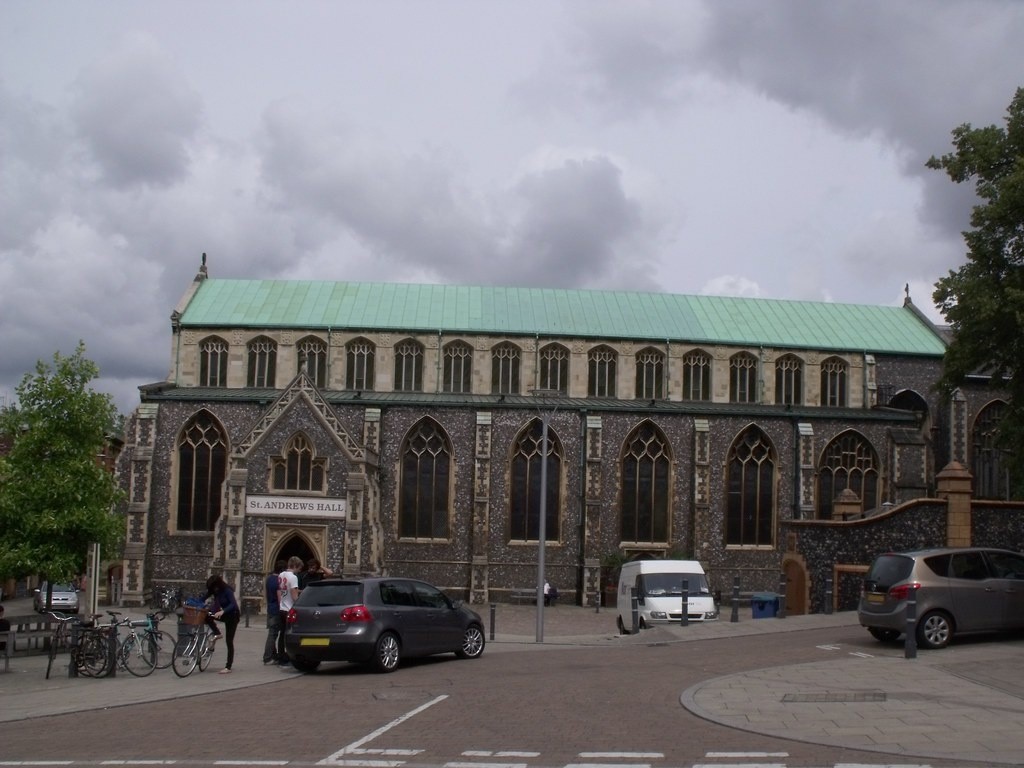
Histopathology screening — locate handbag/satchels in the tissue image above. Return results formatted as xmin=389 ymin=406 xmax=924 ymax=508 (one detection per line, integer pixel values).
xmin=184 ymin=597 xmax=207 ymax=617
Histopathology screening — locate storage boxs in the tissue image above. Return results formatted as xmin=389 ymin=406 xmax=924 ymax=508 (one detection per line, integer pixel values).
xmin=749 ymin=595 xmax=780 ymax=618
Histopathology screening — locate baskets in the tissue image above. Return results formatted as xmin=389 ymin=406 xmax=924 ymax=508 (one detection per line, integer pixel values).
xmin=182 ymin=605 xmax=208 ymax=626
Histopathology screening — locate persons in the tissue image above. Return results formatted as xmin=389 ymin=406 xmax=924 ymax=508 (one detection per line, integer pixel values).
xmin=544 ymin=580 xmax=557 ymax=606
xmin=200 ymin=575 xmax=240 ymax=674
xmin=304 ymin=559 xmax=333 ymax=582
xmin=0 ymin=605 xmax=10 ymax=651
xmin=264 ymin=557 xmax=304 ymax=665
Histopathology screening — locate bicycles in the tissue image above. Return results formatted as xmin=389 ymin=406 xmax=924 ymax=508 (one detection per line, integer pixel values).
xmin=174 ymin=600 xmax=220 ymax=678
xmin=37 ymin=584 xmax=183 ymax=678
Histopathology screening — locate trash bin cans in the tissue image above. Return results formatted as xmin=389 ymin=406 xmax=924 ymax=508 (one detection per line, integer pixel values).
xmin=176 ymin=608 xmax=205 ymax=657
xmin=751 ymin=592 xmax=779 ymax=619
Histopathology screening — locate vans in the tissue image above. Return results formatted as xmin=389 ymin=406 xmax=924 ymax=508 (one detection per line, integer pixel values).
xmin=618 ymin=559 xmax=720 ymax=635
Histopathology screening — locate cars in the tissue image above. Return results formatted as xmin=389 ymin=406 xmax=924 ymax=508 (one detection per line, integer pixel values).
xmin=284 ymin=576 xmax=486 ymax=674
xmin=33 ymin=580 xmax=80 ymax=615
xmin=862 ymin=547 xmax=1024 ymax=650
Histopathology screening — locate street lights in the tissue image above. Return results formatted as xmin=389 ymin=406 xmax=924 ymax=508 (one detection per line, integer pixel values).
xmin=527 ymin=387 xmax=560 ymax=643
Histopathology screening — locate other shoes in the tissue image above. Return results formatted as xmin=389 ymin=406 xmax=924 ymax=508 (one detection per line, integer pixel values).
xmin=219 ymin=668 xmax=232 ymax=673
xmin=210 ymin=634 xmax=223 ymax=641
xmin=263 ymin=653 xmax=294 ymax=669
